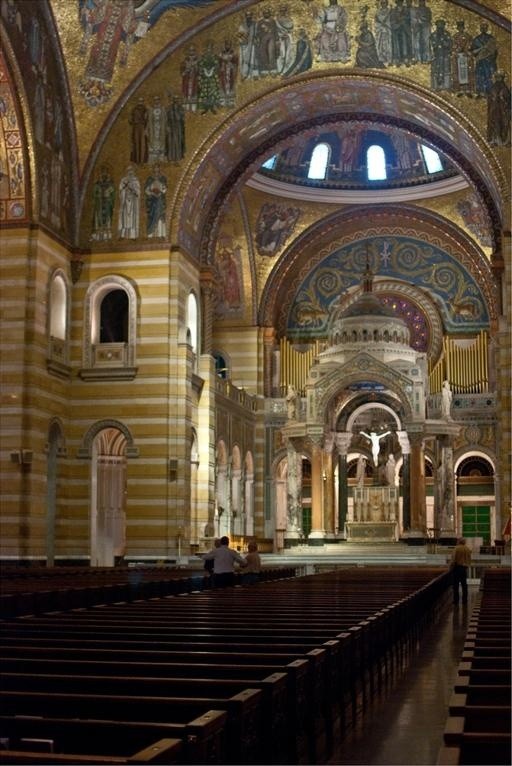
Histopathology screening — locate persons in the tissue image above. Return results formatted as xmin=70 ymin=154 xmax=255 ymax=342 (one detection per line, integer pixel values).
xmin=204 ymin=541 xmax=221 ymax=574
xmin=202 ymin=537 xmax=247 ymax=587
xmin=449 ymin=537 xmax=472 ymax=606
xmin=359 ymin=430 xmax=391 ymax=467
xmin=242 ymin=542 xmax=261 ymax=585
